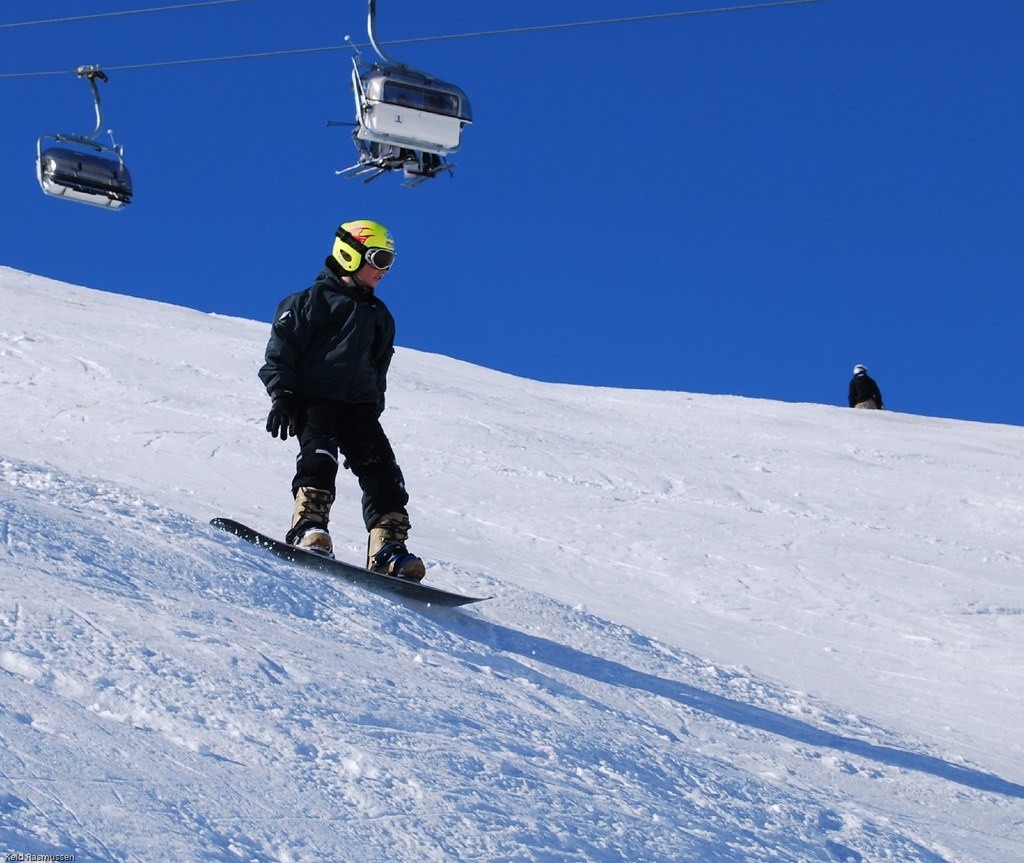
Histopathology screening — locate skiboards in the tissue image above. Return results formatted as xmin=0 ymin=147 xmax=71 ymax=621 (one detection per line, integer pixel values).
xmin=335 ymin=153 xmax=456 ymax=188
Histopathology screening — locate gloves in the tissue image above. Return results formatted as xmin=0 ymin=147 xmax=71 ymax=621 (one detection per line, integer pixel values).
xmin=266 ymin=389 xmax=306 ymax=440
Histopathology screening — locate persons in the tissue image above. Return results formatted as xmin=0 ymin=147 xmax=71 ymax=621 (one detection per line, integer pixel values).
xmin=849 ymin=364 xmax=886 ymax=409
xmin=351 ymin=94 xmax=442 ymax=172
xmin=256 ymin=220 xmax=430 ymax=582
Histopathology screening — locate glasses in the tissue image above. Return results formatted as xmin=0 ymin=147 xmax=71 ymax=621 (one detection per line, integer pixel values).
xmin=335 ymin=227 xmax=397 ymax=270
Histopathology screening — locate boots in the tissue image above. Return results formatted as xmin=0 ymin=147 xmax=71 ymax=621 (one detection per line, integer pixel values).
xmin=367 ymin=512 xmax=425 ymax=581
xmin=292 ymin=486 xmax=333 ymax=554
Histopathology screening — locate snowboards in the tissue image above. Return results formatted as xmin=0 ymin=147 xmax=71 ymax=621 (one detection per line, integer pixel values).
xmin=209 ymin=511 xmax=498 ymax=610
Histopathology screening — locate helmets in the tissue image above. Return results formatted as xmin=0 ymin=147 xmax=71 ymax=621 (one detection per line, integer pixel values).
xmin=333 ymin=220 xmax=395 ymax=272
xmin=854 ymin=365 xmax=867 ymax=374
